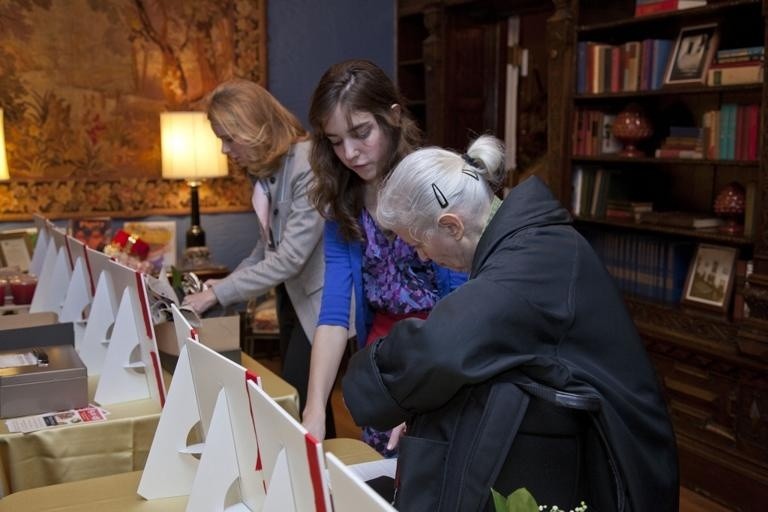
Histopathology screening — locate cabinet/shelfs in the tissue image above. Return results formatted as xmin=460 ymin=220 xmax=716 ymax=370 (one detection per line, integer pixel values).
xmin=555 ymin=6 xmax=768 ymax=512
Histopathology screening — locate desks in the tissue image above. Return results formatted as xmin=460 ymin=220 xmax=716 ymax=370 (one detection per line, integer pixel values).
xmin=0 ymin=437 xmax=390 ymax=512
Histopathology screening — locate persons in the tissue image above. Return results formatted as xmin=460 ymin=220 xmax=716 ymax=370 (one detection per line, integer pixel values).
xmin=297 ymin=55 xmax=474 ymax=463
xmin=696 ymin=254 xmax=729 ymax=291
xmin=335 ymin=134 xmax=683 ymax=512
xmin=678 ymin=34 xmax=706 ymax=72
xmin=178 ymin=78 xmax=356 ymax=442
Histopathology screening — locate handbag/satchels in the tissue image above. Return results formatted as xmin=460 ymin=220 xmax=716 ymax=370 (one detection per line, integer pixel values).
xmin=391 ymin=375 xmax=633 ymax=512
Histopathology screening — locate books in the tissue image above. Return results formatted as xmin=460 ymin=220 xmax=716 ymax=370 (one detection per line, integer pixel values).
xmin=67 ymin=216 xmax=113 ymax=253
xmin=581 ymin=224 xmax=696 ymax=303
xmin=577 ymin=40 xmax=674 ymax=93
xmin=569 ymin=109 xmax=623 ymax=157
xmin=655 ymin=102 xmax=759 ymax=160
xmin=717 ymin=47 xmax=765 ymax=63
xmin=635 ymin=1 xmax=706 ymax=17
xmin=732 ymin=260 xmax=753 ymax=321
xmin=121 ymin=219 xmax=177 ymax=275
xmin=572 ymin=166 xmax=724 ymax=229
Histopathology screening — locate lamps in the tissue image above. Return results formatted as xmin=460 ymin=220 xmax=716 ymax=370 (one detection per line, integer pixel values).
xmin=161 ymin=108 xmax=228 ymax=268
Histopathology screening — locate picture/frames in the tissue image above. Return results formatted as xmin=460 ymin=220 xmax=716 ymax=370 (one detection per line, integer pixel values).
xmin=0 ymin=0 xmax=276 ymax=222
xmin=661 ymin=25 xmax=720 ymax=86
xmin=677 ymin=243 xmax=736 ymax=312
